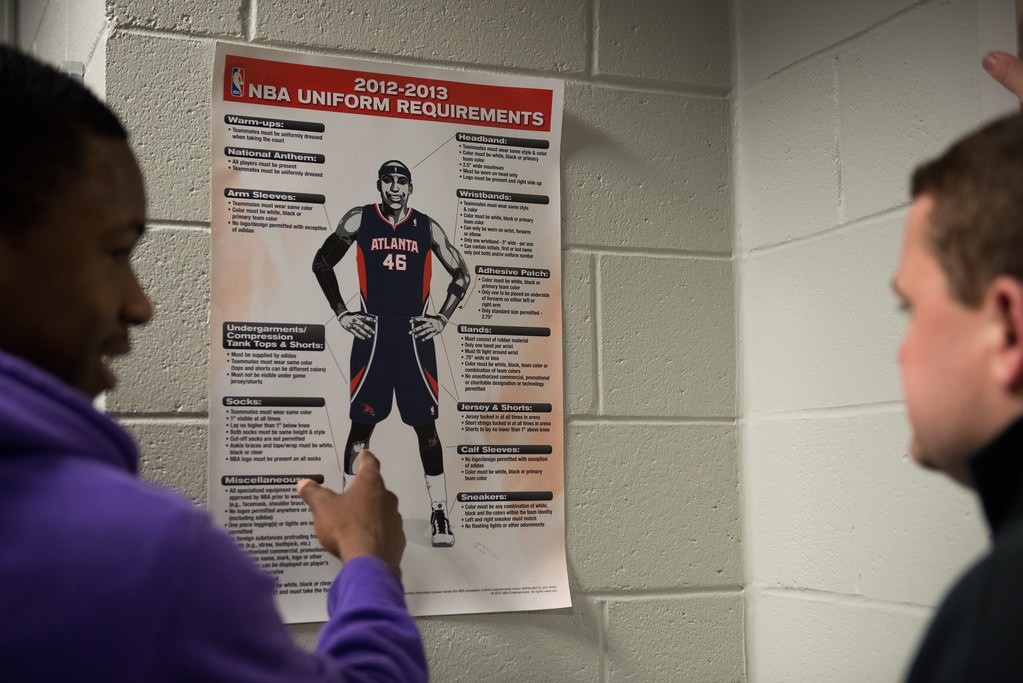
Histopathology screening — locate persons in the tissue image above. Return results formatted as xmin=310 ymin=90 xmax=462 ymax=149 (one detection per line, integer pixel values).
xmin=0 ymin=45 xmax=431 ymax=683
xmin=890 ymin=50 xmax=1023 ymax=683
